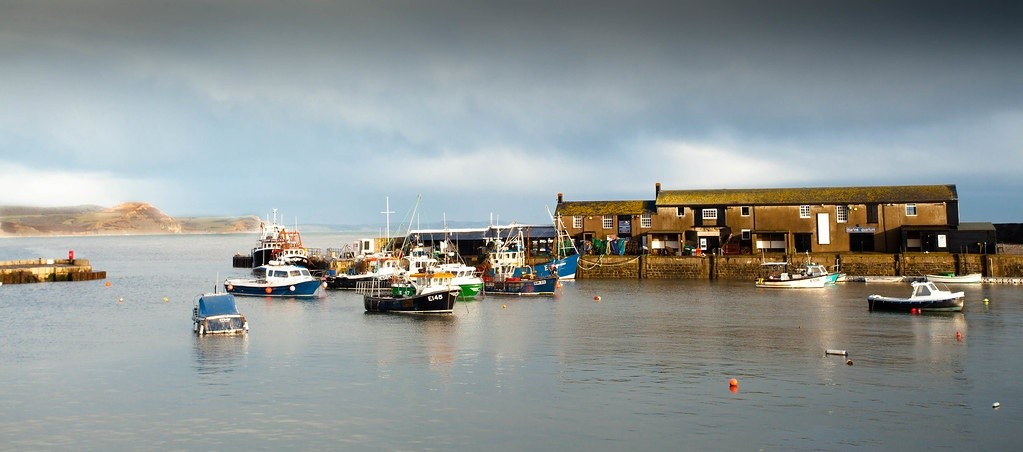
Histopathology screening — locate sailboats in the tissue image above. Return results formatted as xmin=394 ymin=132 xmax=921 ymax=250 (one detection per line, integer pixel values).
xmin=489 ymin=206 xmax=578 ymax=281
xmin=478 ymin=213 xmax=558 ymax=296
xmin=371 ymin=213 xmax=482 ymax=300
xmin=321 ymin=196 xmax=434 ymax=290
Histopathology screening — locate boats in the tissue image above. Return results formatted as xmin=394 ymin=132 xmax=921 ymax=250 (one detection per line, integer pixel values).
xmin=863 ymin=273 xmax=984 ymax=285
xmin=364 ymin=270 xmax=461 ymax=312
xmin=868 ymin=282 xmax=965 ymax=312
xmin=191 ymin=273 xmax=248 ymax=338
xmin=251 ymin=208 xmax=307 ymax=268
xmin=756 ymin=261 xmax=841 ymax=288
xmin=224 ymin=251 xmax=326 ymax=298
